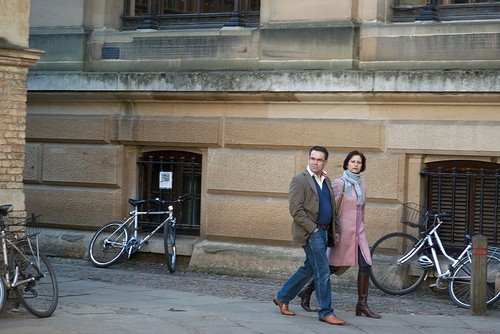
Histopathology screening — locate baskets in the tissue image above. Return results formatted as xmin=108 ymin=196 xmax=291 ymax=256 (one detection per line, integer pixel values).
xmin=400 ymin=202 xmax=427 ymax=229
xmin=7 ymin=212 xmax=43 ymax=242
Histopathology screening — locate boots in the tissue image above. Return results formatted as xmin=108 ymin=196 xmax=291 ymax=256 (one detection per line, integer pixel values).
xmin=356 ymin=272 xmax=381 ymax=318
xmin=299 ymin=279 xmax=315 ymax=311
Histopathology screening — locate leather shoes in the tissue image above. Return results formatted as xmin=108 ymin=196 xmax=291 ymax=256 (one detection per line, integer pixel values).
xmin=319 ymin=313 xmax=346 ymax=324
xmin=272 ymin=296 xmax=295 ymax=315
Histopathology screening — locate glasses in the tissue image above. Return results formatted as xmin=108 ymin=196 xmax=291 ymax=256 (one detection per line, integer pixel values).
xmin=309 ymin=157 xmax=326 ymax=162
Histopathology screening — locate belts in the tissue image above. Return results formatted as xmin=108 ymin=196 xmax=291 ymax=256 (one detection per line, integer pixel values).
xmin=316 ymin=224 xmax=330 ymax=230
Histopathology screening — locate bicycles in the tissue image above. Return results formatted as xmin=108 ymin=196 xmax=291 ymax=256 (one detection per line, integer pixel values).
xmin=369 ymin=201 xmax=500 ymax=307
xmin=0 ymin=203 xmax=59 ymax=318
xmin=88 ymin=191 xmax=194 ymax=273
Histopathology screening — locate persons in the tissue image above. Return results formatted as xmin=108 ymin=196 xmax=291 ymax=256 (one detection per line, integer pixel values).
xmin=298 ymin=151 xmax=381 ymax=319
xmin=273 ymin=145 xmax=346 ymax=325
